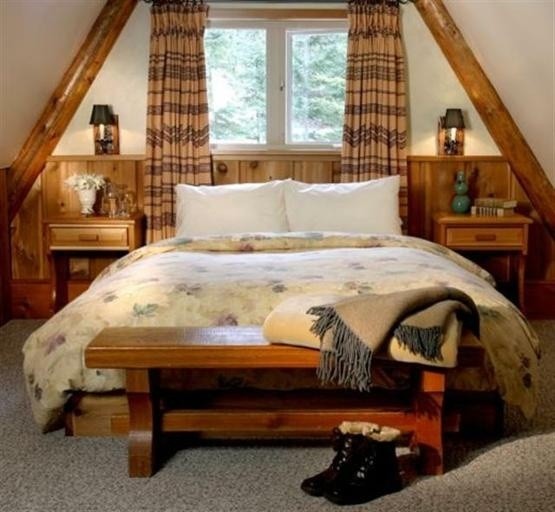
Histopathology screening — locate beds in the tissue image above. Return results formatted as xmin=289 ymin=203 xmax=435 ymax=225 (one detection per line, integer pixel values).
xmin=65 ymin=231 xmax=500 ymax=438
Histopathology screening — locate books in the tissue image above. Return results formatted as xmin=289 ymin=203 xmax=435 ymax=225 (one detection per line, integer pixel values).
xmin=475 ymin=197 xmax=519 ymax=209
xmin=470 ymin=205 xmax=514 ymax=217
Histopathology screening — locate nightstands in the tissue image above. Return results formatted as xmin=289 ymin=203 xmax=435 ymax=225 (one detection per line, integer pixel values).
xmin=42 ymin=212 xmax=145 ymax=317
xmin=430 ymin=210 xmax=534 ymax=317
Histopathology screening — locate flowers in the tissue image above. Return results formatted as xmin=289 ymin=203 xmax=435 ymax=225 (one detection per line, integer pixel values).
xmin=65 ymin=174 xmax=106 ymax=191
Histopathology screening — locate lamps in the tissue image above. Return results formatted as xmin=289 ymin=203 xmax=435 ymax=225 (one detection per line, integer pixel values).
xmin=438 ymin=108 xmax=465 ymax=155
xmin=89 ymin=104 xmax=119 ymax=155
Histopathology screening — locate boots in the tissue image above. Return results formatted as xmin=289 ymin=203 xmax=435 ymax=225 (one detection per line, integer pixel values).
xmin=301 ymin=420 xmax=403 ymax=505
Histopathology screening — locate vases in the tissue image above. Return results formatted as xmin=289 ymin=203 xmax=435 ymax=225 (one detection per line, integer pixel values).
xmin=78 ymin=188 xmax=97 ymax=215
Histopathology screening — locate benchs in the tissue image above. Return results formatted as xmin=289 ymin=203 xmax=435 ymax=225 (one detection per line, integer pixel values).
xmin=85 ymin=327 xmax=486 ymax=477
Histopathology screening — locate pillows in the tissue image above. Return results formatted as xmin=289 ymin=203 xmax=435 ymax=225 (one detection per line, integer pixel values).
xmin=279 ymin=174 xmax=404 ymax=236
xmin=173 ymin=178 xmax=295 ymax=237
